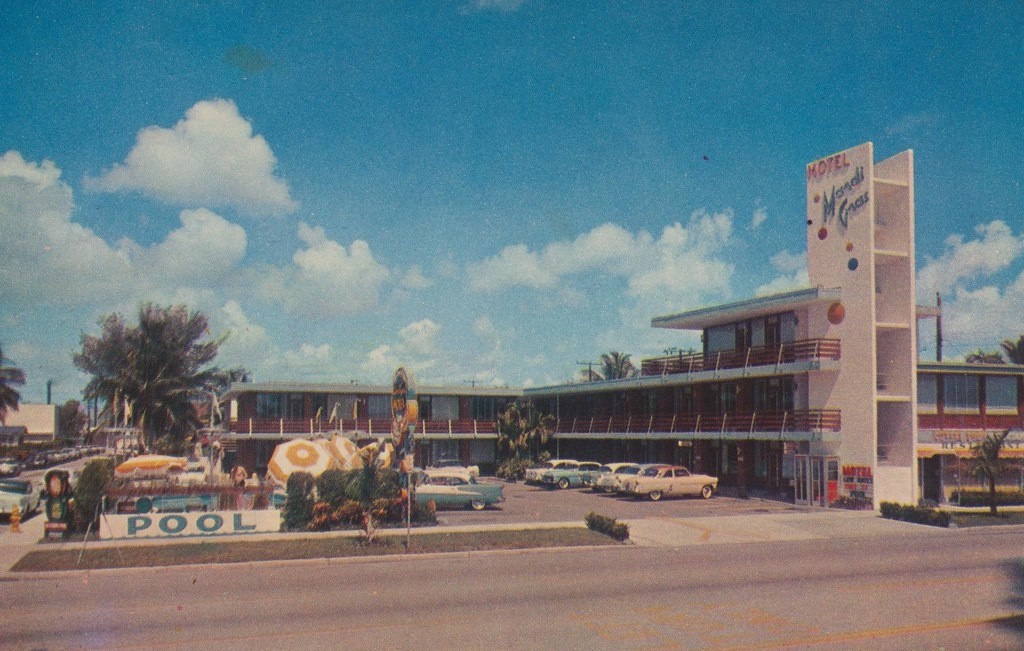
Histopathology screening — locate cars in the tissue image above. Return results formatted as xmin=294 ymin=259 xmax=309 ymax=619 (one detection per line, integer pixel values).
xmin=525 ymin=459 xmax=577 ymax=484
xmin=589 ymin=462 xmax=639 ymax=492
xmin=602 ymin=464 xmax=672 ymax=496
xmin=415 ymin=472 xmax=506 ymax=510
xmin=0 ymin=443 xmax=108 ymax=476
xmin=0 ymin=478 xmax=41 ymax=518
xmin=543 ymin=461 xmax=602 ymax=491
xmin=626 ymin=465 xmax=719 ymax=501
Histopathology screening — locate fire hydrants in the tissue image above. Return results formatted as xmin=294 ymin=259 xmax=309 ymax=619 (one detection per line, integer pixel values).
xmin=10 ymin=505 xmax=22 ymax=534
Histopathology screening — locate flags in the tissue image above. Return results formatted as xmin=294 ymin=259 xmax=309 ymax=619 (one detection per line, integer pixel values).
xmin=315 ymin=402 xmax=358 ymax=425
xmin=112 ymin=395 xmax=132 ymax=426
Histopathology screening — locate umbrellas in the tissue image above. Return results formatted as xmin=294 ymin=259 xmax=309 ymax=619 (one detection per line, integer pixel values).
xmin=115 ymin=454 xmax=189 ymax=487
xmin=267 ymin=435 xmax=360 ymax=491
